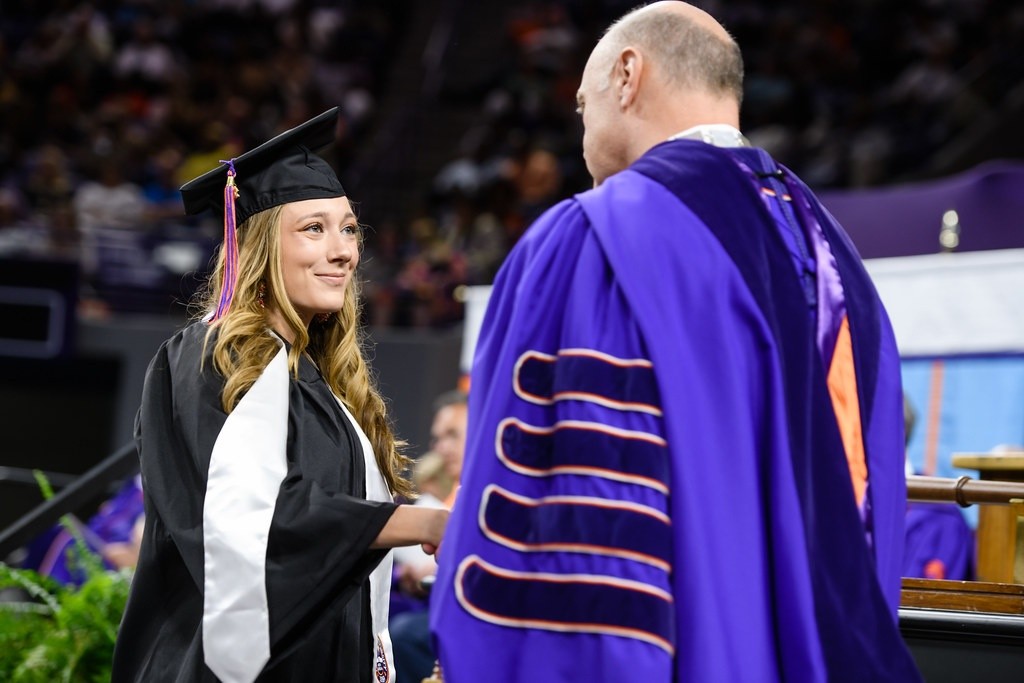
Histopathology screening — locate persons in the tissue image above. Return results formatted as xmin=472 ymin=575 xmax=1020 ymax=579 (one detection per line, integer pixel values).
xmin=423 ymin=0 xmax=929 ymax=683
xmin=113 ymin=104 xmax=453 ymax=682
xmin=386 ymin=389 xmax=469 ymax=681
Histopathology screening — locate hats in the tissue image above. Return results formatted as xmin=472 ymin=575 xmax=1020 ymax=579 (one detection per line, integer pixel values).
xmin=178 ymin=105 xmax=348 ymax=326
